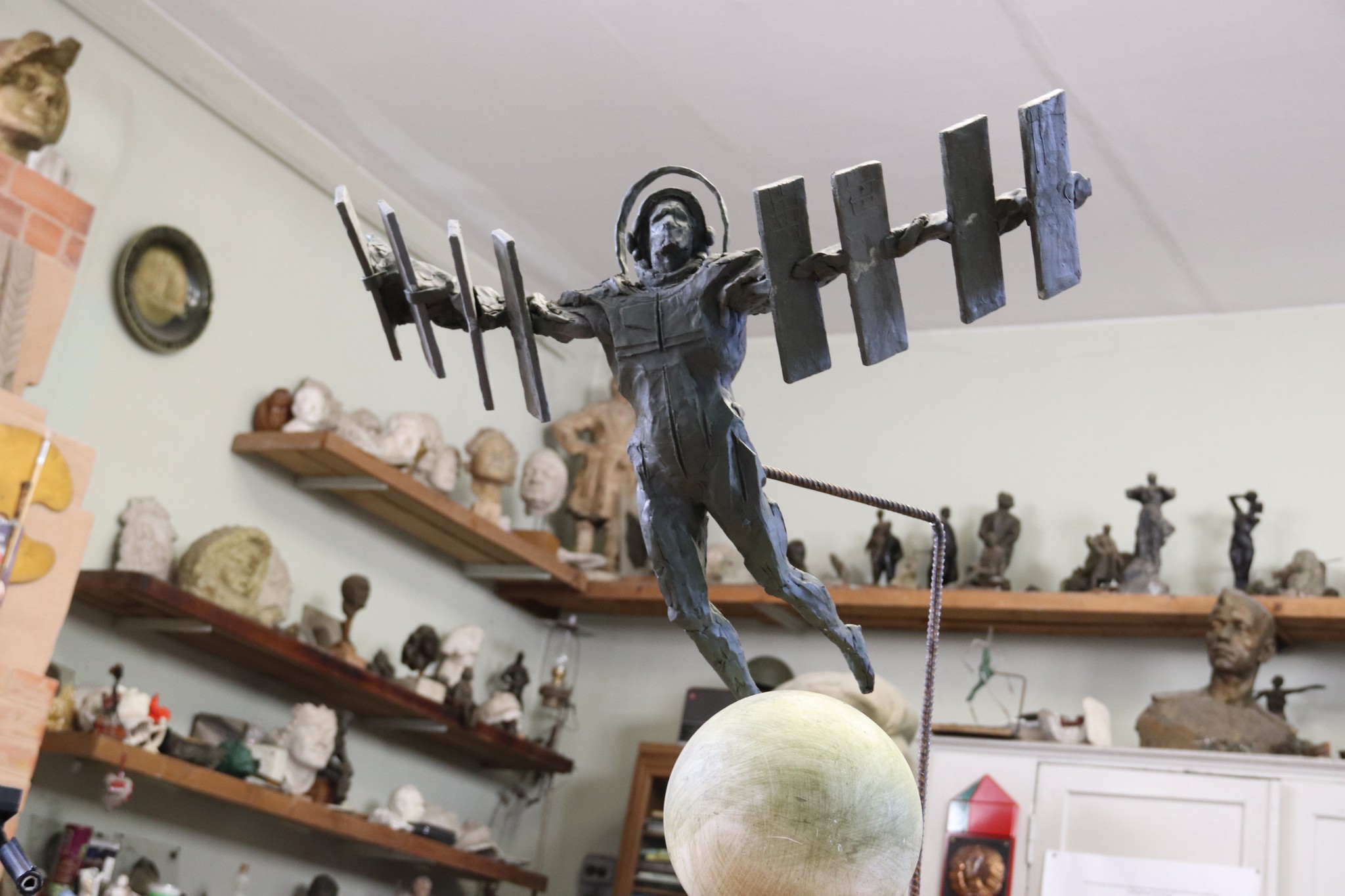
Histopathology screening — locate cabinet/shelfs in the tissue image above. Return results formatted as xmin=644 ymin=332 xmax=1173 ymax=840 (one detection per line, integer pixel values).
xmin=42 ymin=429 xmax=1345 ymax=896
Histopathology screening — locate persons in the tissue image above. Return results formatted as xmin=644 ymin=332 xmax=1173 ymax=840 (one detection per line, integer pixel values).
xmin=551 ymin=379 xmax=640 ymax=576
xmin=341 ymin=576 xmax=371 ymax=618
xmin=520 ymin=446 xmax=569 ymax=520
xmin=866 ymin=508 xmax=903 ymax=587
xmin=1227 ymin=491 xmax=1263 ymax=592
xmin=928 ymin=506 xmax=959 ymax=588
xmin=0 ymin=29 xmax=85 ymax=165
xmin=270 ymin=703 xmax=336 ymax=799
xmin=427 ymin=445 xmax=462 ymax=495
xmin=1251 ymin=674 xmax=1327 ymax=718
xmin=978 ymin=490 xmax=1020 ymax=579
xmin=373 ymin=170 xmax=1093 ymax=698
xmin=500 ymin=650 xmax=530 ymax=737
xmin=447 ymin=667 xmax=476 ymax=725
xmin=1138 ymin=587 xmax=1330 ymax=757
xmin=463 ymin=426 xmax=519 ymax=507
xmin=1125 ymin=471 xmax=1176 ymax=574
xmin=1089 ymin=524 xmax=1123 ymax=592
